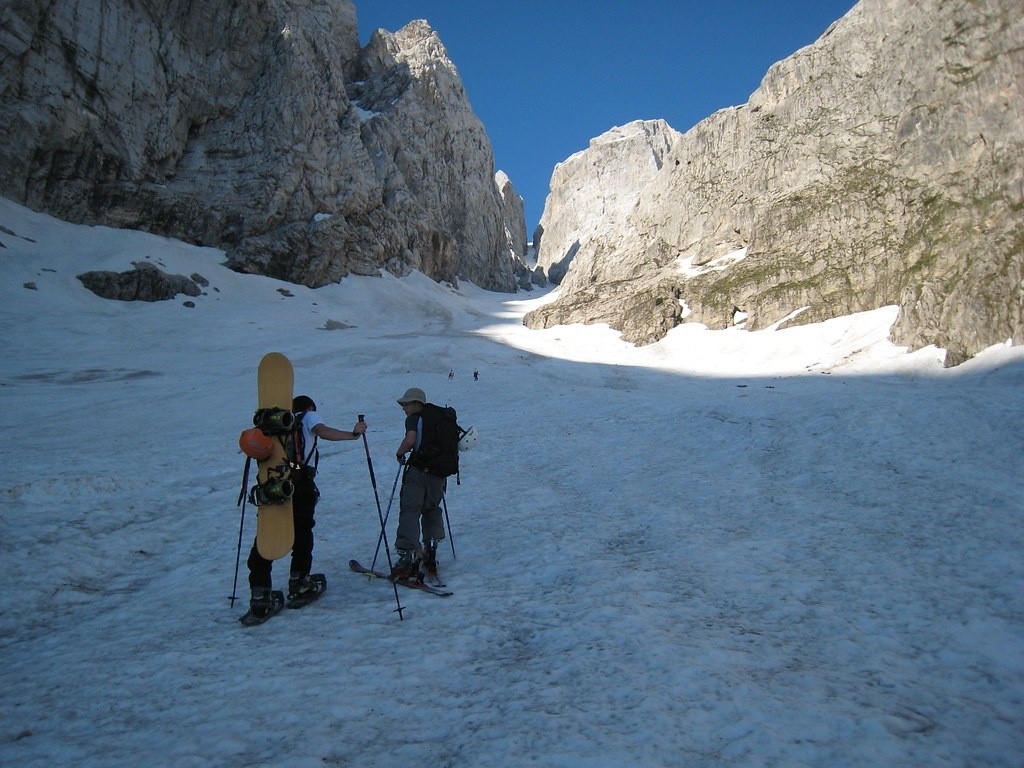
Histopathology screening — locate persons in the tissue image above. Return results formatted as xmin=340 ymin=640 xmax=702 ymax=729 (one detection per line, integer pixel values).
xmin=447 ymin=369 xmax=455 ymax=380
xmin=394 ymin=388 xmax=447 ymax=574
xmin=247 ymin=395 xmax=367 ymax=615
xmin=473 ymin=370 xmax=480 ymax=382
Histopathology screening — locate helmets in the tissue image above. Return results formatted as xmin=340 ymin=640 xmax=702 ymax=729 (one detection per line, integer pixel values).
xmin=458 ymin=425 xmax=478 ymax=452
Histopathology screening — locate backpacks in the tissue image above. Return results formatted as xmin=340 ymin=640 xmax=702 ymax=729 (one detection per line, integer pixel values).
xmin=411 ymin=402 xmax=461 ymax=486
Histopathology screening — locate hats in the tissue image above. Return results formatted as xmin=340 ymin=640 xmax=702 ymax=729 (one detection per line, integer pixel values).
xmin=397 ymin=388 xmax=425 ymax=406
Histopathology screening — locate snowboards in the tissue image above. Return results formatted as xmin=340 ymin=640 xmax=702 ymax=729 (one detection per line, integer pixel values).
xmin=255 ymin=351 xmax=295 ymax=561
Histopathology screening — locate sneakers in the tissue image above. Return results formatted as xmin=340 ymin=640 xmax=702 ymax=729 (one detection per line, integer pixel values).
xmin=423 ymin=540 xmax=438 ymax=571
xmin=391 ymin=557 xmax=420 ymax=574
xmin=289 ymin=571 xmax=318 ymax=597
xmin=248 ymin=589 xmax=275 ymax=615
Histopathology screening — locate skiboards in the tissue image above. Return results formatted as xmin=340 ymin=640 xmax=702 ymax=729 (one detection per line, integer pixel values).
xmin=350 ymin=559 xmax=453 ymax=600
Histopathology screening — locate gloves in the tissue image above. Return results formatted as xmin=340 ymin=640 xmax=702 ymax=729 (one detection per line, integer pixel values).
xmin=397 ymin=453 xmax=406 ymax=466
